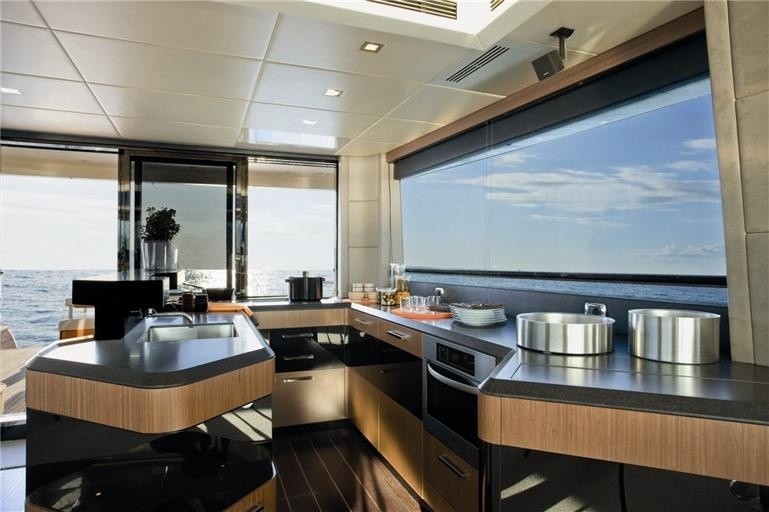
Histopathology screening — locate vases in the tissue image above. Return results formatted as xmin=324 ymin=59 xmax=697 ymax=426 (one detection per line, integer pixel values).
xmin=167 ymin=244 xmax=178 ymax=271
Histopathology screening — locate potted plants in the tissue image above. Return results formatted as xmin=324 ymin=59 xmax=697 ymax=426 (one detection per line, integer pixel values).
xmin=137 ymin=206 xmax=180 ymax=271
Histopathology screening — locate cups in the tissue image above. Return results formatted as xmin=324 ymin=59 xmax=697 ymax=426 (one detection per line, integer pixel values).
xmin=399 ymin=295 xmax=442 ymax=314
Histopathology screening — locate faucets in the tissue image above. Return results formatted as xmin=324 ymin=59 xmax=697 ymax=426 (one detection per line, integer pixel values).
xmin=145 ymin=307 xmax=196 ymax=329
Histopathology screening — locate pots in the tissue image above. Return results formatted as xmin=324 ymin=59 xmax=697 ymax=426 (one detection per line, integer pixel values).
xmin=284 ymin=276 xmax=325 ymax=302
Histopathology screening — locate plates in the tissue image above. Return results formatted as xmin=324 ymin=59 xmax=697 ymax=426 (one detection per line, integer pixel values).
xmin=449 ymin=302 xmax=508 ymax=328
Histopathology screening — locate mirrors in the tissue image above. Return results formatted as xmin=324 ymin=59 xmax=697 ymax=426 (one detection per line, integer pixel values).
xmin=135 ymin=155 xmax=234 ymax=299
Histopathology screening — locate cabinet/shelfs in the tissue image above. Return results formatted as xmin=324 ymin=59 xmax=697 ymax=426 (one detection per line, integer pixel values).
xmin=346 ymin=309 xmax=378 ymax=449
xmin=378 ymin=318 xmax=423 ymax=501
xmin=423 ymin=431 xmax=479 ymax=512
xmin=253 ymin=309 xmax=346 ymax=427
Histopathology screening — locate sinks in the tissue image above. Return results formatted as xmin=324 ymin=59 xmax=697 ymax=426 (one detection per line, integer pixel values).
xmin=136 ymin=322 xmax=238 ymax=343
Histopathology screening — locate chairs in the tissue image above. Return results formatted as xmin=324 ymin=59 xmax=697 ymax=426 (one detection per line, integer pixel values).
xmin=58 ymin=298 xmax=95 ymax=339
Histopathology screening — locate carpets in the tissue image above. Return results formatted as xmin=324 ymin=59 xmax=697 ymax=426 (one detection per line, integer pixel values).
xmin=0 ymin=438 xmax=27 ymax=469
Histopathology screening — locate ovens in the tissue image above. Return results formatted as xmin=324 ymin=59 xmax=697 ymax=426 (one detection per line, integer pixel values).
xmin=422 ymin=332 xmax=496 ymax=471
